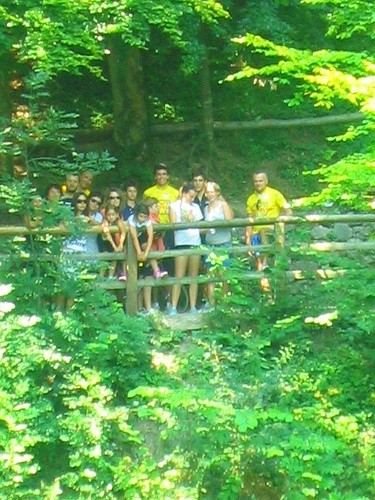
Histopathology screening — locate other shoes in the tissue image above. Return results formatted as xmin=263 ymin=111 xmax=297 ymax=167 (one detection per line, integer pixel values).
xmin=190 ymin=309 xmax=198 ymax=314
xmin=119 ymin=273 xmax=127 ymax=281
xmin=154 ymin=271 xmax=168 ymax=279
xmin=169 ymin=308 xmax=177 ymax=316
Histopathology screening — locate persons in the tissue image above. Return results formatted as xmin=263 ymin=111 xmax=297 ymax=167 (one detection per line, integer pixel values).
xmin=245 ymin=172 xmax=292 ymax=304
xmin=22 ymin=163 xmax=235 ymax=317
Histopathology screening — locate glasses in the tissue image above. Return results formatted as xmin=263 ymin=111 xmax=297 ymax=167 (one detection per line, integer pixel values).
xmin=91 ymin=198 xmax=101 ymax=206
xmin=109 ymin=196 xmax=120 ymax=200
xmin=77 ymin=200 xmax=86 ymax=204
